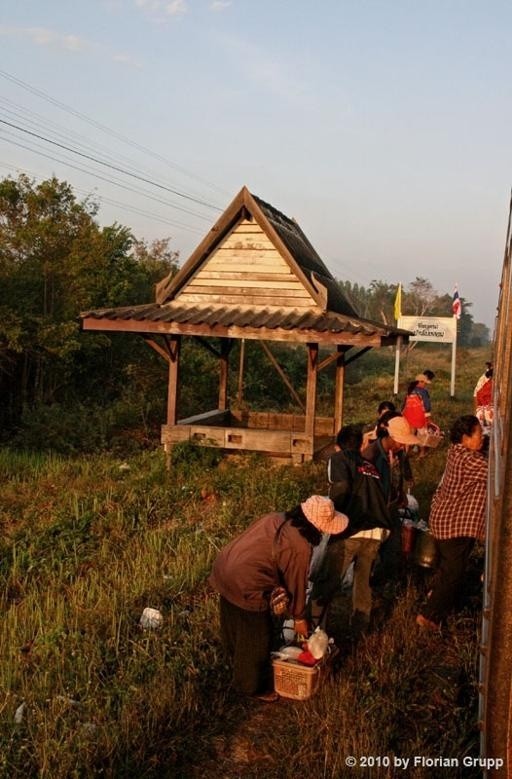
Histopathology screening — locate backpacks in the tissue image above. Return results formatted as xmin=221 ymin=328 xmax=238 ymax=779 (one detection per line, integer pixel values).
xmin=402 ymin=394 xmax=427 ymax=429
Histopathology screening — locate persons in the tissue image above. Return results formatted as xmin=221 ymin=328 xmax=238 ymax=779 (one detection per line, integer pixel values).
xmin=415 ymin=415 xmax=489 ymax=633
xmin=206 ymin=495 xmax=349 ymax=703
xmin=306 ymin=370 xmax=440 ymax=634
xmin=473 ymin=362 xmax=493 ymax=451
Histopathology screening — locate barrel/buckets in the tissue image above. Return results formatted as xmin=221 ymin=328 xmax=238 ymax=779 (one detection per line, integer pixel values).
xmin=400 ymin=506 xmax=416 ymax=554
xmin=415 ymin=528 xmax=440 ymax=572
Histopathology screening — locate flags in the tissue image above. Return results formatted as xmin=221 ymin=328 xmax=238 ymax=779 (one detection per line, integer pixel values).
xmin=452 ymin=283 xmax=461 ymax=320
xmin=394 ymin=282 xmax=401 ymax=320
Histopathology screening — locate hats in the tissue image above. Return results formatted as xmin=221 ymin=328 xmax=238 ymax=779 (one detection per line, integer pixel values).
xmin=414 ymin=373 xmax=432 ymax=384
xmin=300 ymin=494 xmax=349 ymax=536
xmin=385 ymin=416 xmax=421 ymax=445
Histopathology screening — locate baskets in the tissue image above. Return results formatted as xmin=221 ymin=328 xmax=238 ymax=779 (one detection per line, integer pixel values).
xmin=271 ymin=638 xmax=340 ymax=701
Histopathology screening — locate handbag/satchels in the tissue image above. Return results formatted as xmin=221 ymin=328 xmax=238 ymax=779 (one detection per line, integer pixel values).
xmin=271 ymin=586 xmax=290 ymax=616
